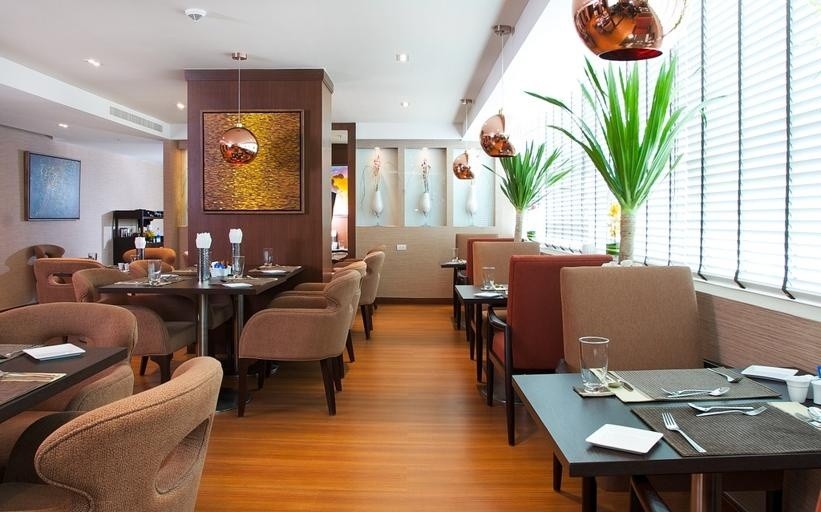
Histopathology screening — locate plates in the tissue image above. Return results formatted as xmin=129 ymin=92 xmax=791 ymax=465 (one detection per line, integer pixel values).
xmin=474 ymin=291 xmax=503 ymax=299
xmin=585 ymin=422 xmax=664 ymax=454
xmin=21 ymin=341 xmax=87 ymax=361
xmin=741 ymin=364 xmax=801 ymax=383
xmin=221 ymin=283 xmax=254 ymax=289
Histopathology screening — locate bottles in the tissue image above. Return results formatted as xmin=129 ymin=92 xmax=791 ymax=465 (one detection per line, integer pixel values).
xmin=156 ymin=228 xmax=161 ymax=243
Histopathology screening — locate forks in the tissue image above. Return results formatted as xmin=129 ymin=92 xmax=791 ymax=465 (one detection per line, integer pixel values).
xmin=695 ymin=404 xmax=767 ymax=417
xmin=660 ymin=387 xmax=714 ymax=394
xmin=1 ymin=343 xmax=34 ymax=359
xmin=708 ymin=366 xmax=743 ymax=383
xmin=794 ymin=412 xmax=821 ymax=426
xmin=662 ymin=412 xmax=708 ymax=454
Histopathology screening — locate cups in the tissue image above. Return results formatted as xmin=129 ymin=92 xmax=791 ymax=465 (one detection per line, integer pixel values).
xmin=579 ymin=336 xmax=610 ymax=392
xmin=147 ymin=259 xmax=163 ymax=284
xmin=233 ymin=256 xmax=246 ymax=278
xmin=452 ymin=248 xmax=459 ymax=262
xmin=211 ymin=265 xmax=231 ymax=277
xmin=118 ymin=263 xmax=129 ymax=271
xmin=263 ymin=248 xmax=274 ymax=266
xmin=785 ymin=373 xmax=821 ymax=404
xmin=88 ymin=252 xmax=97 ymax=260
xmin=482 ymin=266 xmax=496 ymax=289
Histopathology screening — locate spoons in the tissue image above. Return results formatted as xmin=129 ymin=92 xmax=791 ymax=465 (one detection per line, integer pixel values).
xmin=688 ymin=402 xmax=754 ymax=412
xmin=807 ymin=406 xmax=821 ymax=422
xmin=668 ymin=386 xmax=731 ymax=398
xmin=597 ymin=369 xmax=624 ymax=388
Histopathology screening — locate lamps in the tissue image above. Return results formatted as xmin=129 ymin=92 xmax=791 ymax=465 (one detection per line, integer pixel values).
xmin=217 ymin=48 xmax=260 ymax=166
xmin=479 ymin=20 xmax=526 ymax=159
xmin=567 ymin=0 xmax=689 ymax=62
xmin=452 ymin=95 xmax=485 ymax=180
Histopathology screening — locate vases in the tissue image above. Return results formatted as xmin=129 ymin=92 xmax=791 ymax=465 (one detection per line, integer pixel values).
xmin=464 ymin=185 xmax=479 ymax=218
xmin=370 ymin=191 xmax=383 ymax=216
xmin=418 ymin=191 xmax=431 ymax=216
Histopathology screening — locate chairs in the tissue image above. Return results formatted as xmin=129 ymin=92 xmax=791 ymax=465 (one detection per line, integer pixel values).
xmin=332 ymin=245 xmax=386 ymax=339
xmin=27 ymin=244 xmax=176 ymax=283
xmin=73 ymin=267 xmax=197 ymax=385
xmin=1 ymin=356 xmax=223 ymax=511
xmin=128 ymin=260 xmax=235 ymax=356
xmin=2 ymin=302 xmax=138 ymax=411
xmin=238 ymin=270 xmax=360 ymax=418
xmin=31 ymin=258 xmax=108 ymax=304
xmin=439 ymin=229 xmax=821 ymax=512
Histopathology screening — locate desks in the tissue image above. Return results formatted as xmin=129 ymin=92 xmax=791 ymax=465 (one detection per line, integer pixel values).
xmin=2 ymin=343 xmax=129 ymax=425
xmin=175 ymin=265 xmax=302 ymax=281
xmin=99 ymin=274 xmax=287 ymax=411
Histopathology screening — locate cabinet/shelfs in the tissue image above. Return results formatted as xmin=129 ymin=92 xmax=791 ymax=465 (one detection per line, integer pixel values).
xmin=109 ymin=208 xmax=164 ymax=267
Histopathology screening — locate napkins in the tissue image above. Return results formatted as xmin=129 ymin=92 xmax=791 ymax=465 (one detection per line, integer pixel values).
xmin=223 ymin=283 xmax=251 ymax=287
xmin=263 ymin=269 xmax=286 ymax=273
xmin=23 ymin=343 xmax=85 ymax=361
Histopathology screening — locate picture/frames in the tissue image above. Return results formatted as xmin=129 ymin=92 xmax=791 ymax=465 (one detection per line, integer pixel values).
xmin=197 ymin=106 xmax=309 ymax=214
xmin=22 ymin=149 xmax=82 ymax=222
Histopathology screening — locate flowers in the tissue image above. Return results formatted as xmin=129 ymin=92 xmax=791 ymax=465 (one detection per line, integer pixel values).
xmin=415 ymin=156 xmax=431 ymax=191
xmin=368 ymin=151 xmax=386 ymax=190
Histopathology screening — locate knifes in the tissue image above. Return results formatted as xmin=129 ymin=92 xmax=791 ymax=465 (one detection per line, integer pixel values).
xmin=608 ymin=370 xmax=634 ymax=391
